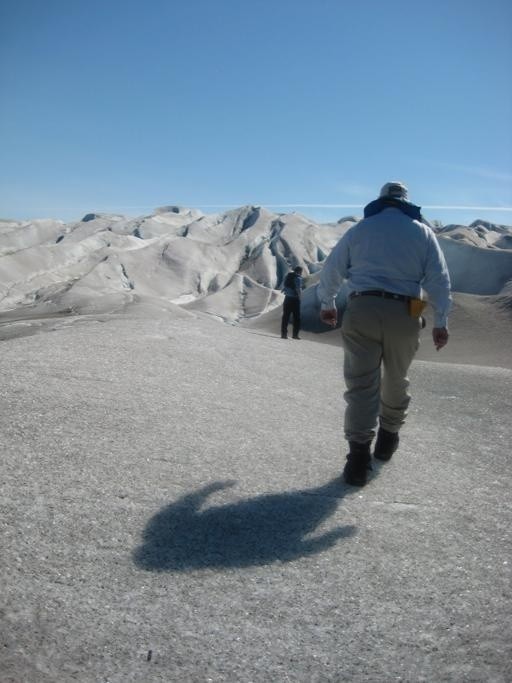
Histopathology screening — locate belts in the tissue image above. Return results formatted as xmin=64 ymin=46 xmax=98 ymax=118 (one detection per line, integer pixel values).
xmin=349 ymin=290 xmax=412 ymax=301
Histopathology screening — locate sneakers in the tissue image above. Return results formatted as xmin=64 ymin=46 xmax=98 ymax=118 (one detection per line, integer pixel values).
xmin=373 ymin=425 xmax=400 ymax=463
xmin=343 ymin=440 xmax=373 ymax=487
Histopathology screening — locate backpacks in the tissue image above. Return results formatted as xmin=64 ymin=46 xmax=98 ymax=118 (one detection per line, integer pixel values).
xmin=284 ymin=271 xmax=301 ymax=289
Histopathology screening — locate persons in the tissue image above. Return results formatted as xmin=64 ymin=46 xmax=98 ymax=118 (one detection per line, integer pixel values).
xmin=279 ymin=265 xmax=308 ymax=339
xmin=317 ymin=181 xmax=453 ymax=486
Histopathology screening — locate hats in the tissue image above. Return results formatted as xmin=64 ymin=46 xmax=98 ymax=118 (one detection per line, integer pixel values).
xmin=362 ymin=180 xmax=422 ymax=219
